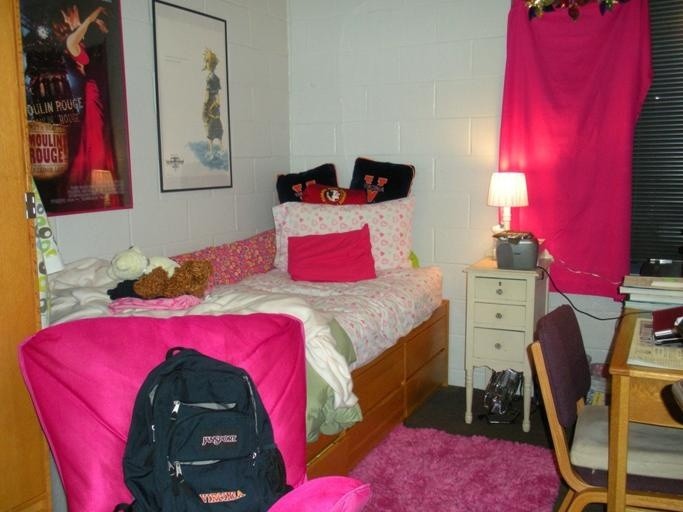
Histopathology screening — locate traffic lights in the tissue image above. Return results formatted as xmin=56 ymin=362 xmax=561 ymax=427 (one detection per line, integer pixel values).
xmin=266 ymin=475 xmax=372 ymax=512
xmin=271 ymin=157 xmax=416 ymax=282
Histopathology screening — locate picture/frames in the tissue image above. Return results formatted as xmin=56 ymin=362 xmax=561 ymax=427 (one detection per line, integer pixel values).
xmin=606 ymin=308 xmax=683 ymax=512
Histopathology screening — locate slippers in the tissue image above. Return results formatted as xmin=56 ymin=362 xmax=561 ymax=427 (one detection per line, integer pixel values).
xmin=114 ymin=345 xmax=293 ymax=512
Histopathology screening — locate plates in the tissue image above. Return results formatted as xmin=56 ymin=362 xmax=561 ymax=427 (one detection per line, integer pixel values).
xmin=462 ymin=257 xmax=546 ymax=432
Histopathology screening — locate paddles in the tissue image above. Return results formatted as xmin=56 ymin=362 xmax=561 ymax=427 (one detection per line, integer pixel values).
xmin=347 ymin=422 xmax=564 ymax=512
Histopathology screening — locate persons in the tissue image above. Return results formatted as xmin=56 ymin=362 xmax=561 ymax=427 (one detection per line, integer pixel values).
xmin=50 ymin=3 xmax=119 ymax=207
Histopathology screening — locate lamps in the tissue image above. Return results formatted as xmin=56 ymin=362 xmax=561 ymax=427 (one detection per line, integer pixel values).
xmin=17 ymin=312 xmax=373 ymax=512
xmin=529 ymin=304 xmax=683 ymax=512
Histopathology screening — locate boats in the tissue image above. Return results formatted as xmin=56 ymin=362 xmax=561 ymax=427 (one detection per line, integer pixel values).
xmin=497 ymin=237 xmax=538 ymax=270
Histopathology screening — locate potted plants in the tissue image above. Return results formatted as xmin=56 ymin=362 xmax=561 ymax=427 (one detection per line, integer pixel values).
xmin=152 ymin=0 xmax=233 ymax=192
xmin=14 ymin=0 xmax=134 ymax=216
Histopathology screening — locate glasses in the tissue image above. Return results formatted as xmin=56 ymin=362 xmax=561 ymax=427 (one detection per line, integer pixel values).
xmin=488 ymin=172 xmax=529 ymax=260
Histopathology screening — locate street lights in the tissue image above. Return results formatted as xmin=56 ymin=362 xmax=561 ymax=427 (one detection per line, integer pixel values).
xmin=481 ymin=370 xmax=539 ymax=417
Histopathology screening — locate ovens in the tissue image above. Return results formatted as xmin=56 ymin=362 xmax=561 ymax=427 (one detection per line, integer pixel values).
xmin=47 ymin=228 xmax=449 ymax=512
